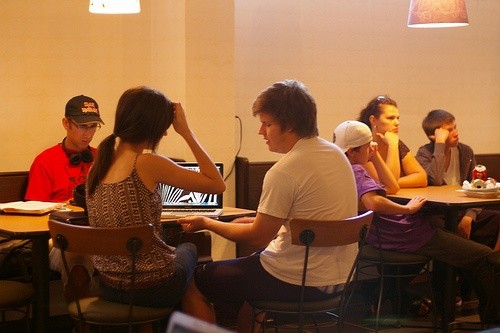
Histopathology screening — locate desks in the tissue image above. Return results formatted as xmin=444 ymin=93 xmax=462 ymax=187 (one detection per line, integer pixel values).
xmin=0 ymin=202 xmax=84 ymax=333
xmin=161 ymin=206 xmax=256 ymax=247
xmin=386 ymin=185 xmax=500 ymax=333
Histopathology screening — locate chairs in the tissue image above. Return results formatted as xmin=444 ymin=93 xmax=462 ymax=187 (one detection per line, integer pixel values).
xmin=47 ymin=218 xmax=173 ymax=333
xmin=245 ymin=210 xmax=374 ymax=333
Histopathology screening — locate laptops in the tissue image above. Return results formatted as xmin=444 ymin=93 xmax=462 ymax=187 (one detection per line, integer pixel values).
xmin=159 ymin=162 xmax=224 ymax=219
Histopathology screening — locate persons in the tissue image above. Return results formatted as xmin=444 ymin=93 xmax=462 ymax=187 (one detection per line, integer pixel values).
xmin=359 ymin=95 xmax=430 ymax=316
xmin=333 ymin=121 xmax=500 ymax=327
xmin=85 ymin=86 xmax=226 ymax=305
xmin=24 ymin=95 xmax=105 ymax=294
xmin=177 ymin=81 xmax=357 ymax=333
xmin=414 ymin=109 xmax=500 ymax=305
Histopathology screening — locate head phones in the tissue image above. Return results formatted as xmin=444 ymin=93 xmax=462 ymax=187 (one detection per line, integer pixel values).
xmin=62 ymin=136 xmax=93 ymax=165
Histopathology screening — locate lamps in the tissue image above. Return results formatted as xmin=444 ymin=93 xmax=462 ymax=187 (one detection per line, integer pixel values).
xmin=89 ymin=0 xmax=140 ymax=15
xmin=408 ymin=0 xmax=469 ymax=29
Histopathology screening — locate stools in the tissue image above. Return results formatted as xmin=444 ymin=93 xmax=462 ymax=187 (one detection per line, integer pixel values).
xmin=342 ymin=245 xmax=438 ymax=333
xmin=0 ymin=278 xmax=38 ymax=333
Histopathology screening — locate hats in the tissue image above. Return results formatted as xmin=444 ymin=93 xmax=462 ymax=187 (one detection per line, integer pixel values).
xmin=65 ymin=95 xmax=104 ymax=124
xmin=332 ymin=120 xmax=372 ymax=153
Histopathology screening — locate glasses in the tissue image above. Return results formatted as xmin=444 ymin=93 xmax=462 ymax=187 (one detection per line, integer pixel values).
xmin=68 ymin=117 xmax=102 ymax=133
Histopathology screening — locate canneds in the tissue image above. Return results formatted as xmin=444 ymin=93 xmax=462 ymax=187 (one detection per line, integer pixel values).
xmin=471 ymin=164 xmax=496 ymax=189
